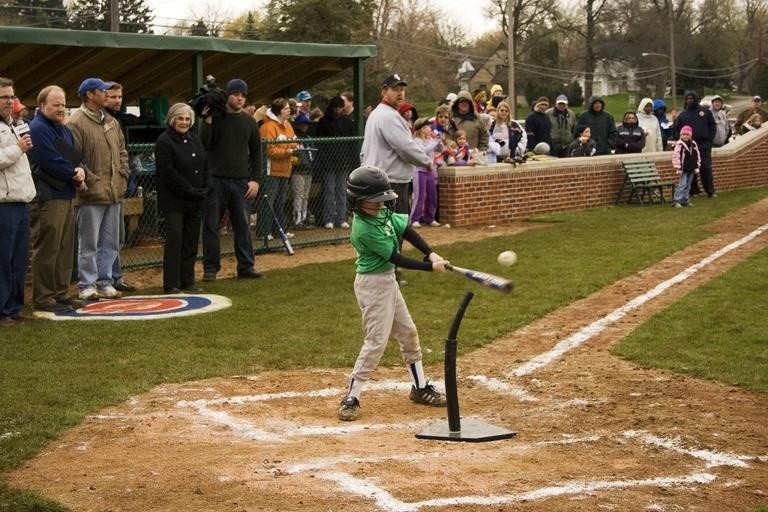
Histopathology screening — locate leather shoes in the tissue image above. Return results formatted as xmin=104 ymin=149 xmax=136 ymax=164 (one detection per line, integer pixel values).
xmin=203 ymin=275 xmax=216 ymax=282
xmin=0 ymin=319 xmax=16 ymax=327
xmin=62 ymin=298 xmax=86 ymax=308
xmin=12 ymin=314 xmax=27 ymax=323
xmin=115 ymin=282 xmax=138 ymax=292
xmin=164 ymin=289 xmax=185 ymax=295
xmin=37 ymin=302 xmax=73 ymax=313
xmin=184 ymin=287 xmax=203 ymax=292
xmin=238 ymin=268 xmax=263 ymax=279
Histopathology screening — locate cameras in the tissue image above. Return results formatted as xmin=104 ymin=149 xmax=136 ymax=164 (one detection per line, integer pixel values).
xmin=188 ymin=75 xmax=231 ymax=122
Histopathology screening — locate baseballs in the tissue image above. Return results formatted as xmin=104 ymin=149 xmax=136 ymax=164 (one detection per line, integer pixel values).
xmin=497 ymin=251 xmax=516 ymax=267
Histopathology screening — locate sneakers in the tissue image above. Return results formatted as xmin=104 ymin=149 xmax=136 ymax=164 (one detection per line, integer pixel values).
xmin=78 ymin=288 xmax=100 ymax=300
xmin=292 ymin=223 xmax=306 ymax=231
xmin=98 ymin=287 xmax=123 ymax=298
xmin=673 ymin=202 xmax=683 ymax=209
xmin=682 ymin=202 xmax=693 ymax=208
xmin=340 ymin=222 xmax=350 ymax=229
xmin=285 ymin=232 xmax=295 ymax=240
xmin=428 ymin=220 xmax=441 ymax=227
xmin=409 ymin=381 xmax=448 ymax=408
xmin=709 ymin=193 xmax=718 ymax=198
xmin=412 ymin=222 xmax=422 ymax=228
xmin=338 ymin=396 xmax=361 ymax=422
xmin=325 ymin=222 xmax=334 ymax=229
xmin=256 ymin=234 xmax=274 ymax=240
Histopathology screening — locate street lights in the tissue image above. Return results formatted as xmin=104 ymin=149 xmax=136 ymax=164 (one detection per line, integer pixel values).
xmin=642 ymin=51 xmax=676 ymax=111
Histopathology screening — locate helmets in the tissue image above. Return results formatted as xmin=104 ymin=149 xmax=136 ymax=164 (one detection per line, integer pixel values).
xmin=346 ymin=165 xmax=399 ymax=226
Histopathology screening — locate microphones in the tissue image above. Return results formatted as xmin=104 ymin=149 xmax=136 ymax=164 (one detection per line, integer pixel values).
xmin=13 ymin=119 xmax=33 ymax=146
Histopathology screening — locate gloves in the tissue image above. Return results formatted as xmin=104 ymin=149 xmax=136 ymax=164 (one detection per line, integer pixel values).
xmin=695 ymin=169 xmax=700 ymax=175
xmin=675 ymin=169 xmax=682 ymax=175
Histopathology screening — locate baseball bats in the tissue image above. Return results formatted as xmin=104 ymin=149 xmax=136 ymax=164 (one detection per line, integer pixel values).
xmin=424 ymin=256 xmax=514 ymax=293
xmin=264 ymin=189 xmax=295 ymax=256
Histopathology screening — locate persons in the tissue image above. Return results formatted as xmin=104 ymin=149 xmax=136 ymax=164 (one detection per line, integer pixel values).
xmin=428 ymin=103 xmax=457 ymax=145
xmin=295 ymin=90 xmax=323 ymax=224
xmin=198 ymin=79 xmax=265 ymax=283
xmin=671 ymin=91 xmax=717 ymax=198
xmin=241 ymin=96 xmax=267 ymax=226
xmin=443 ymin=129 xmax=475 ymax=166
xmin=408 ymin=116 xmax=440 ymax=228
xmin=488 ymin=102 xmax=527 ymax=163
xmin=671 ymin=126 xmax=702 ymax=209
xmin=155 ymin=103 xmax=216 ymax=295
xmin=545 ymin=95 xmax=579 ymax=158
xmin=571 ymin=96 xmax=621 ymax=156
xmin=614 ymin=112 xmax=646 ymax=154
xmin=28 ymin=85 xmax=89 ymax=314
xmin=0 ymin=77 xmax=37 ymax=328
xmin=64 ymin=78 xmax=132 ymax=305
xmin=479 ymin=113 xmax=501 ymax=163
xmin=11 ymin=95 xmax=26 ymax=128
xmin=314 ymin=97 xmax=359 ymax=229
xmin=734 ymin=95 xmax=768 ymax=139
xmin=482 ymin=84 xmax=502 ymax=113
xmin=491 ymin=95 xmax=507 ymax=109
xmin=339 ymin=91 xmax=368 ymax=126
xmin=397 ymin=102 xmax=419 ymax=137
xmin=430 ymin=98 xmax=458 ymax=139
xmin=290 ymin=114 xmax=320 ymax=231
xmin=428 ymin=92 xmax=457 ymax=121
xmin=360 ymin=74 xmax=434 ymax=288
xmin=255 ymin=98 xmax=303 ymax=241
xmin=336 ymin=164 xmax=449 ymax=422
xmin=471 ymin=88 xmax=488 ymax=113
xmin=485 ymin=109 xmax=496 ymax=117
xmin=101 ymin=81 xmax=137 ymax=292
xmin=740 ymin=114 xmax=762 ymax=136
xmin=288 ymin=99 xmax=302 ymax=126
xmin=450 ymin=90 xmax=490 ymax=156
xmin=635 ymin=98 xmax=663 ymax=153
xmin=652 ymin=100 xmax=670 ymax=152
xmin=524 ymin=96 xmax=553 ymax=157
xmin=708 ymin=94 xmax=729 ymax=148
xmin=362 ymin=103 xmax=372 ymax=117
xmin=530 ymin=101 xmax=538 ymax=112
xmin=567 ymin=125 xmax=597 ymax=157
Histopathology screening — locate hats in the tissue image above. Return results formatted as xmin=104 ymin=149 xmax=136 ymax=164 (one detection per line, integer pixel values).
xmin=12 ymin=97 xmax=26 ymax=113
xmin=380 ymin=73 xmax=408 ymax=88
xmin=331 ymin=96 xmax=345 ymax=107
xmin=493 ymin=95 xmax=507 ymax=108
xmin=680 ymin=125 xmax=692 ymax=137
xmin=753 ymin=96 xmax=761 ymax=99
xmin=473 ymin=89 xmax=486 ymax=103
xmin=78 ymin=77 xmax=113 ymax=95
xmin=491 ymin=84 xmax=503 ymax=96
xmin=297 ymin=90 xmax=312 ymax=102
xmin=224 ymin=78 xmax=249 ymax=98
xmin=575 ymin=126 xmax=586 ymax=137
xmin=536 ymin=96 xmax=550 ymax=107
xmin=413 ymin=116 xmax=429 ymax=130
xmin=556 ymin=94 xmax=568 ymax=105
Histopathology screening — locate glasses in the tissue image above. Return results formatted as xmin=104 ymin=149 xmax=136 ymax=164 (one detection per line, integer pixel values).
xmin=754 ymin=99 xmax=761 ymax=102
xmin=499 ymin=109 xmax=509 ymax=112
xmin=88 ymin=88 xmax=106 ymax=93
xmin=0 ymin=95 xmax=17 ymax=100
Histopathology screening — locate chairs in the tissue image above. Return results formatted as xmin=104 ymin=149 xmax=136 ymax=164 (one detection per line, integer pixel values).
xmin=616 ymin=159 xmax=677 ymax=208
